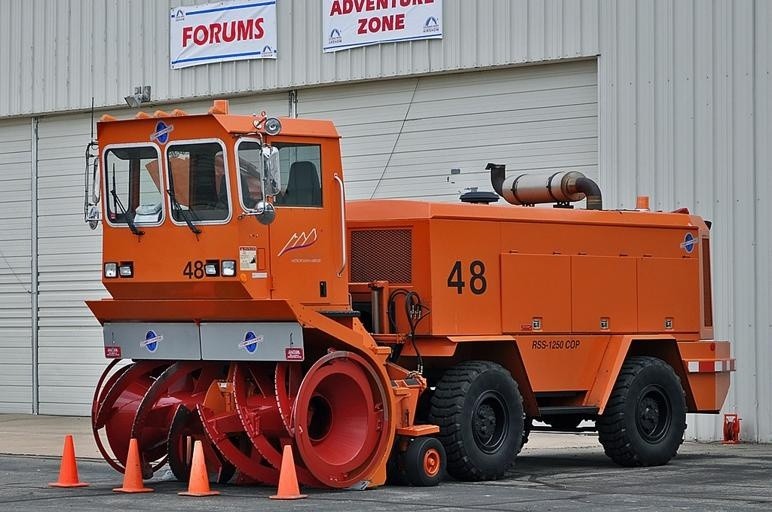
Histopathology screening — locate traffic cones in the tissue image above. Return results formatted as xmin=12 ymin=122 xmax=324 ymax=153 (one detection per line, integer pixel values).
xmin=175 ymin=441 xmax=220 ymax=498
xmin=46 ymin=435 xmax=87 ymax=489
xmin=112 ymin=439 xmax=154 ymax=495
xmin=267 ymin=445 xmax=309 ymax=501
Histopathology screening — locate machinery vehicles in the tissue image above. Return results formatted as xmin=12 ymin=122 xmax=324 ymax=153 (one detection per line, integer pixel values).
xmin=78 ymin=94 xmax=740 ymax=494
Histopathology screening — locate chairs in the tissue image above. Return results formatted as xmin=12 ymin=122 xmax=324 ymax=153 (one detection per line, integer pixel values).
xmin=212 ymin=173 xmax=250 ymax=212
xmin=280 ymin=160 xmax=321 ymax=207
